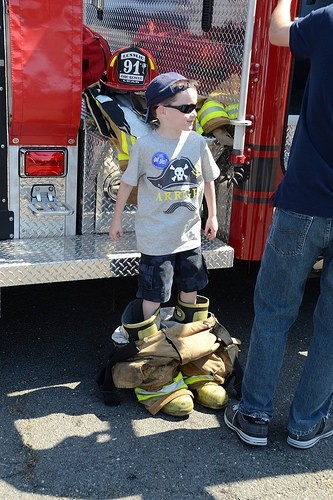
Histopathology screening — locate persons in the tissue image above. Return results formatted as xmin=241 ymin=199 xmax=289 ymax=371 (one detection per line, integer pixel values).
xmin=224 ymin=0 xmax=333 ymax=449
xmin=109 ymin=72 xmax=220 ymax=321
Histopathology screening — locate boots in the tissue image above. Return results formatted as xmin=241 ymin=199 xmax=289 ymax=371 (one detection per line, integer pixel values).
xmin=172 ymin=295 xmax=228 ymax=409
xmin=120 ymin=299 xmax=193 ymax=415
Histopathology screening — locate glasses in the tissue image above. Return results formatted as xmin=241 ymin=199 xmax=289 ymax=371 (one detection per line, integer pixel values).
xmin=152 ymin=104 xmax=196 ymax=113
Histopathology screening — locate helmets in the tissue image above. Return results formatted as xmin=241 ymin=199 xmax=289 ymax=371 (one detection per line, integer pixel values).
xmin=99 ymin=47 xmax=157 ymax=91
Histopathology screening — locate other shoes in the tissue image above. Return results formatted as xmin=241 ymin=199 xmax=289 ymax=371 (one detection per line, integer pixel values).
xmin=223 ymin=404 xmax=267 ymax=445
xmin=287 ymin=410 xmax=332 ymax=448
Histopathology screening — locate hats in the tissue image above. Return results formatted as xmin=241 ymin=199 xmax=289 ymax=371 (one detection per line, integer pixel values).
xmin=144 ymin=72 xmax=189 ymax=123
